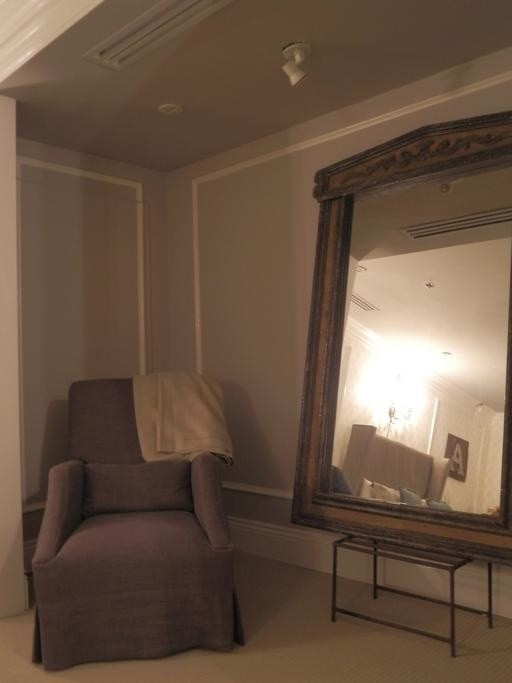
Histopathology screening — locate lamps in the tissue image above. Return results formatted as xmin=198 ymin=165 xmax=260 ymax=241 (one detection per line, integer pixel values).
xmin=280 ymin=41 xmax=313 ymax=88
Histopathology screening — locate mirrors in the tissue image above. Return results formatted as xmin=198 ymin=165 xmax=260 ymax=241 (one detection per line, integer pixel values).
xmin=288 ymin=110 xmax=509 ymax=569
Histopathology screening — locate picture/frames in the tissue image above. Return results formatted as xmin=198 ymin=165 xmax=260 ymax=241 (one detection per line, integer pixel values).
xmin=444 ymin=433 xmax=468 ymax=483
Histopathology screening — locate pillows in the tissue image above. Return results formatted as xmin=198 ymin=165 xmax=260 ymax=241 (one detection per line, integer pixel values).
xmin=355 ymin=478 xmax=451 ymax=514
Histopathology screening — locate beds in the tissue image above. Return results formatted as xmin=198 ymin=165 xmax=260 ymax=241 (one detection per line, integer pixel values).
xmin=344 ymin=424 xmax=452 ymax=512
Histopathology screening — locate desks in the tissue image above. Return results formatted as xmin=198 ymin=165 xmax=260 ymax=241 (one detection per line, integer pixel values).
xmin=328 ymin=533 xmax=496 ymax=660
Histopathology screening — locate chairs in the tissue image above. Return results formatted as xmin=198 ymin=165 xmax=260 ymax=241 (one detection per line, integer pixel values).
xmin=23 ymin=377 xmax=243 ymax=672
